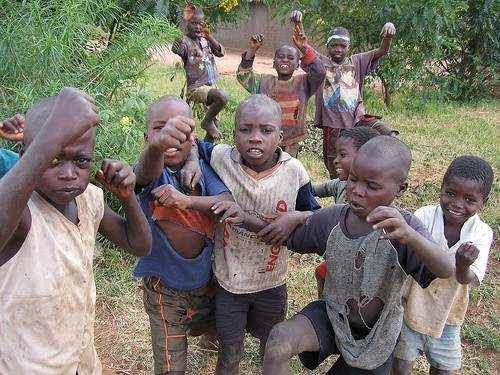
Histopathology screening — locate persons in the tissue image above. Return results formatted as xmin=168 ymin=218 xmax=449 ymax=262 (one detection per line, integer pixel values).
xmin=0 ymin=84 xmax=153 ymax=375
xmin=210 ymin=134 xmax=454 ymax=375
xmin=176 ymin=93 xmax=323 ymax=375
xmin=171 ymin=3 xmax=229 ymax=146
xmin=390 ymin=151 xmax=495 ymax=375
xmin=309 ymin=125 xmax=384 ymax=303
xmin=288 ymin=7 xmax=396 ymax=180
xmin=0 ymin=113 xmax=25 ymax=179
xmin=129 ymin=91 xmax=237 ymax=375
xmin=235 ymin=24 xmax=328 ymax=164
